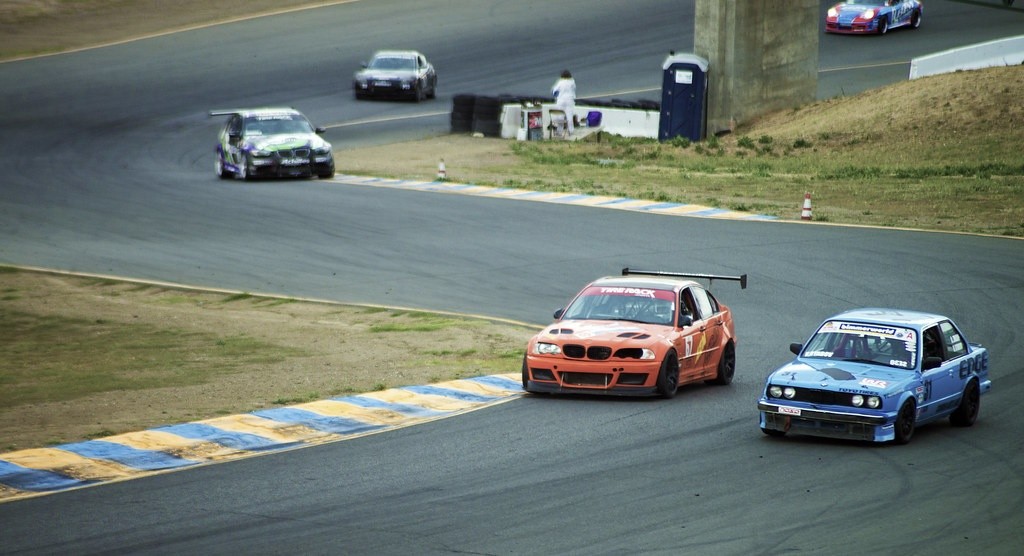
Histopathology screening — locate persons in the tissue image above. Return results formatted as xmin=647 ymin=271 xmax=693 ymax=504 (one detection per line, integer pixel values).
xmin=680 ymin=299 xmax=692 ymax=318
xmin=552 ymin=70 xmax=575 ymax=135
xmin=923 ymin=330 xmax=938 ymax=362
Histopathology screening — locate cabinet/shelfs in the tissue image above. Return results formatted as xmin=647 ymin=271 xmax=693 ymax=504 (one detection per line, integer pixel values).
xmin=520 ymin=110 xmax=542 ymax=142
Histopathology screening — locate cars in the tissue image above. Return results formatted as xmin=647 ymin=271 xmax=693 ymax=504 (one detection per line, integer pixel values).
xmin=354 ymin=50 xmax=438 ymax=103
xmin=756 ymin=306 xmax=993 ymax=445
xmin=209 ymin=105 xmax=336 ymax=183
xmin=522 ymin=267 xmax=748 ymax=399
xmin=824 ymin=0 xmax=924 ymax=36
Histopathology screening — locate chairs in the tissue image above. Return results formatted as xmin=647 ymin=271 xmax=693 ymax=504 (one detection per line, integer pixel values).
xmin=592 ymin=297 xmax=621 ymax=316
xmin=548 ymin=110 xmax=567 ymax=140
xmin=585 ymin=111 xmax=602 ymax=143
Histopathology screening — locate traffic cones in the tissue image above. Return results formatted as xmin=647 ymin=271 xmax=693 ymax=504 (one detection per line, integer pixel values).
xmin=436 ymin=158 xmax=447 ymax=181
xmin=798 ymin=194 xmax=814 ymax=221
